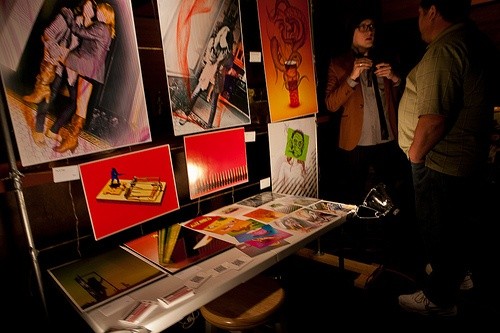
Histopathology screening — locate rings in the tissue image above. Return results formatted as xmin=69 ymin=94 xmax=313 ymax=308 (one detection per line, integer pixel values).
xmin=360 ymin=64 xmax=362 ymax=66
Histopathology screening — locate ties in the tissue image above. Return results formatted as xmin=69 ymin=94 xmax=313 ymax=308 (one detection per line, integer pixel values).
xmin=372 ymin=67 xmax=389 ymax=141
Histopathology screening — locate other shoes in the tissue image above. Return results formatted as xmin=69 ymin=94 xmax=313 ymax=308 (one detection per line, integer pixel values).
xmin=398 ymin=290 xmax=437 ymax=311
xmin=426 ymin=263 xmax=473 ymax=291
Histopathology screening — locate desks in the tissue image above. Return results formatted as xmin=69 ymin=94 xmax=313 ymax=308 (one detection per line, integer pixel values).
xmin=47 ymin=192 xmax=359 ymax=333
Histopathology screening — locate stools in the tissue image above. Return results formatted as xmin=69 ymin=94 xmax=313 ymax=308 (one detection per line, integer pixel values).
xmin=199 ymin=273 xmax=285 ymax=333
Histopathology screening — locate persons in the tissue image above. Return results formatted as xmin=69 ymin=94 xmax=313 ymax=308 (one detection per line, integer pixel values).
xmin=395 ymin=0 xmax=497 ymax=317
xmin=325 ymin=11 xmax=402 ymax=151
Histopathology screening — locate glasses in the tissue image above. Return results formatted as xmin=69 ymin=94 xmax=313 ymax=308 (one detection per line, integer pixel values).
xmin=354 ymin=22 xmax=376 ymax=33
